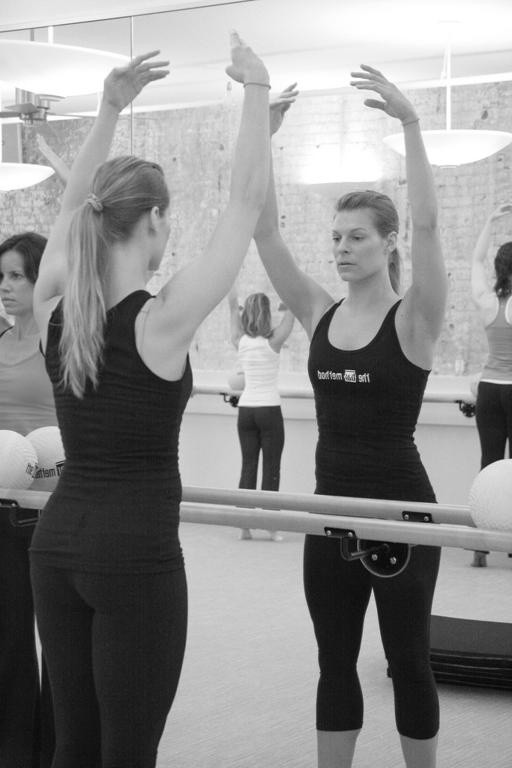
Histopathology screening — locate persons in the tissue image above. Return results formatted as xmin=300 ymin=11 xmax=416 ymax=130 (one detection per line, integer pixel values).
xmin=0 ymin=228 xmax=61 ymax=766
xmin=26 ymin=24 xmax=281 ymax=766
xmin=468 ymin=200 xmax=512 ymax=569
xmin=252 ymin=63 xmax=448 ymax=766
xmin=227 ymin=282 xmax=294 ymax=544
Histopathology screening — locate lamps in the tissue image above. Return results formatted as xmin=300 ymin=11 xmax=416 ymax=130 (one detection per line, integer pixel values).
xmin=377 ymin=42 xmax=511 ymax=175
